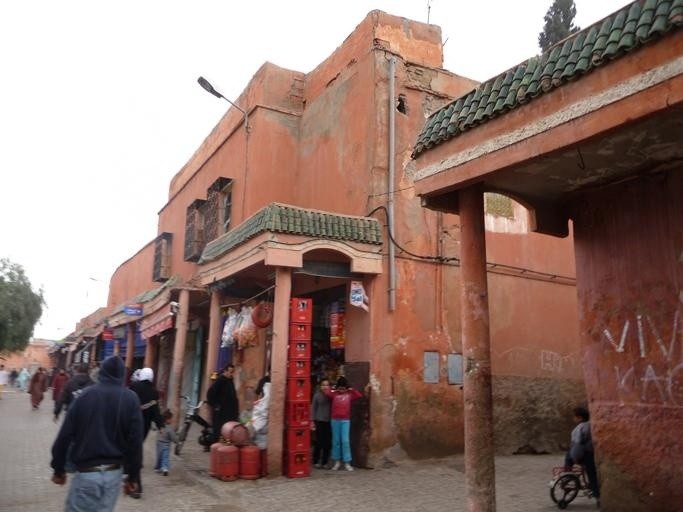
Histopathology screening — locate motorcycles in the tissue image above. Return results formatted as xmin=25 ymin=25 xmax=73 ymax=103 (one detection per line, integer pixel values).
xmin=173 ymin=394 xmax=214 ymax=457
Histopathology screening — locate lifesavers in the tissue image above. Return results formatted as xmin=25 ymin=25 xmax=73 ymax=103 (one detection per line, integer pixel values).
xmin=252 ymin=302 xmax=273 ymax=327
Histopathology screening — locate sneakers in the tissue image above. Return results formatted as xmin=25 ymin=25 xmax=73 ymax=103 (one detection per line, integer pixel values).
xmin=121 ymin=476 xmax=143 ymax=499
xmin=155 ymin=466 xmax=169 ymax=476
xmin=312 ymin=460 xmax=353 ymax=473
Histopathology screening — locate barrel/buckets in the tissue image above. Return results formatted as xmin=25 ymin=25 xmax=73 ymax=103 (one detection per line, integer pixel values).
xmin=210 ymin=438 xmax=241 ymax=480
xmin=221 ymin=421 xmax=251 ymax=443
xmin=240 ymin=445 xmax=261 ymax=479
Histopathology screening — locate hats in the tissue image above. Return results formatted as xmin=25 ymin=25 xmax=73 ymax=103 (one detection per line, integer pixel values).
xmin=102 ymin=354 xmax=125 ymax=382
xmin=130 ymin=366 xmax=155 ymax=383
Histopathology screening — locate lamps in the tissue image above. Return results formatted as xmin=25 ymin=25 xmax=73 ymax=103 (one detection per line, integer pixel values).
xmin=198 ymin=76 xmax=248 ymax=128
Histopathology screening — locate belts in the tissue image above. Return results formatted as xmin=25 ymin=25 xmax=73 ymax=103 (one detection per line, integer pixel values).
xmin=78 ymin=463 xmax=123 ymax=473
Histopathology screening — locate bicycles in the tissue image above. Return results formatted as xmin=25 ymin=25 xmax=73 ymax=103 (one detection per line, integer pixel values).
xmin=549 ymin=462 xmax=600 ymax=508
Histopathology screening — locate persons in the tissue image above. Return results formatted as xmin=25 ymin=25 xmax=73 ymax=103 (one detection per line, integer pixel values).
xmin=154 ymin=409 xmax=181 ymax=476
xmin=50 ymin=355 xmax=143 ymax=512
xmin=253 ymin=364 xmax=270 ymax=403
xmin=122 ymin=366 xmax=166 ymax=475
xmin=0 ymin=361 xmax=103 ymax=424
xmin=563 ymin=406 xmax=598 ymax=499
xmin=323 ymin=376 xmax=363 ymax=472
xmin=310 ymin=378 xmax=332 ymax=470
xmin=206 ymin=363 xmax=239 ymax=444
